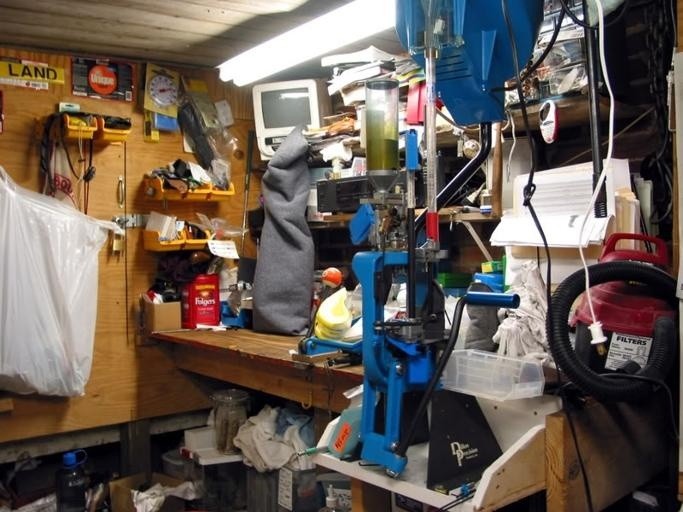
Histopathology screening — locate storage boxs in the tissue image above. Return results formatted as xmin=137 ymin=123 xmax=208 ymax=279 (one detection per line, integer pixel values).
xmin=143 ymin=302 xmax=182 ymax=331
xmin=181 ymin=425 xmax=247 ymax=512
xmin=277 ymin=458 xmax=324 ymax=511
xmin=538 ymin=39 xmax=586 ymax=71
xmin=404 ymin=80 xmax=430 ymax=125
xmin=547 ymin=63 xmax=590 ymax=96
xmin=315 ymin=470 xmax=352 ymax=500
xmin=108 ymin=471 xmax=189 ymax=511
xmin=160 ymin=445 xmax=196 ymax=480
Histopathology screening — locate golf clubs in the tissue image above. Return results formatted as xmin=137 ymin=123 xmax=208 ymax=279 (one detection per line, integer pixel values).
xmin=238 ymin=131 xmax=255 ymax=291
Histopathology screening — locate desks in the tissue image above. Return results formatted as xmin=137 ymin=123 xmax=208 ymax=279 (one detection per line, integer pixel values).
xmin=153 ymin=319 xmax=363 ymax=415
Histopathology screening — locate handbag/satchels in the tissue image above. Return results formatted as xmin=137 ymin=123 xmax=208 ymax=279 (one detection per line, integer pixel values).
xmin=40 ymin=141 xmax=81 ymax=210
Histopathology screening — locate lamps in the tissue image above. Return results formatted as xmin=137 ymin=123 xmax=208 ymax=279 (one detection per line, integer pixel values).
xmin=214 ymin=1 xmax=404 ymax=87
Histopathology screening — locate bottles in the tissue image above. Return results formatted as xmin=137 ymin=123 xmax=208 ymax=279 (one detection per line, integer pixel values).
xmin=54 ymin=448 xmax=88 ymax=512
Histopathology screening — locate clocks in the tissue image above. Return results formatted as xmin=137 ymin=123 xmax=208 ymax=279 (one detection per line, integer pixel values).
xmin=148 ymin=74 xmax=178 ymax=107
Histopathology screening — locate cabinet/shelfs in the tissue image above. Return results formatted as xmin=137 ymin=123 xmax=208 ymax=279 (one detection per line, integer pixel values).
xmin=64 ymin=113 xmax=235 ymax=252
xmin=314 ymin=376 xmax=666 ymax=512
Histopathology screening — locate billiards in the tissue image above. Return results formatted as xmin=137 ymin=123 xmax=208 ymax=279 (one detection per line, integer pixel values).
xmin=322 ymin=268 xmax=341 ymax=288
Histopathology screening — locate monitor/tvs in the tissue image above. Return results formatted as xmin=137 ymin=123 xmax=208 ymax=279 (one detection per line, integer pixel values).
xmin=251 ymin=77 xmax=334 ymax=163
